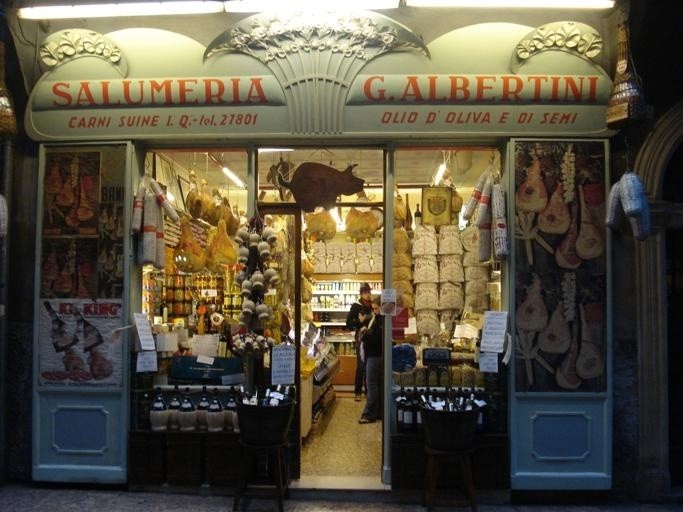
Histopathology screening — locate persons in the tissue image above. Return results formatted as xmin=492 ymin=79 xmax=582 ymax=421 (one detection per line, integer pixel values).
xmin=358 ymin=294 xmax=384 ymax=425
xmin=344 ymin=282 xmax=374 ymax=402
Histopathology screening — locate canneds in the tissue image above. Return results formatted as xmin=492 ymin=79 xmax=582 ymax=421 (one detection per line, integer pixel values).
xmin=162 ymin=271 xmax=242 ymax=321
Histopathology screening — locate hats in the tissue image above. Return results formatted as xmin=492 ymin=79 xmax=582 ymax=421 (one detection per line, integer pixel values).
xmin=358 ymin=282 xmax=373 ymax=292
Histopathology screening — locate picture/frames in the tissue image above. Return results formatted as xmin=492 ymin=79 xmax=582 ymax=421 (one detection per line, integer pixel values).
xmin=421 ymin=186 xmax=453 ymax=225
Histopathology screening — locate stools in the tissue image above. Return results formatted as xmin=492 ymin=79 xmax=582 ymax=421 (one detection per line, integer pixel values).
xmin=422 ymin=446 xmax=476 ymax=512
xmin=423 ymin=362 xmax=452 ymax=388
xmin=233 ymin=439 xmax=289 ymax=512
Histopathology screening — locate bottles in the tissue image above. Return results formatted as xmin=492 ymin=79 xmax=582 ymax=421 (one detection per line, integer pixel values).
xmin=239 ymin=383 xmax=290 ymax=406
xmin=150 ymin=384 xmax=238 ymax=431
xmin=397 ymin=384 xmax=486 ymax=434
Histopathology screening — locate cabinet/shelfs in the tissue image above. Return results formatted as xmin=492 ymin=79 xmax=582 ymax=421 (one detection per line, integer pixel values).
xmin=310 ymin=280 xmax=382 ymax=384
